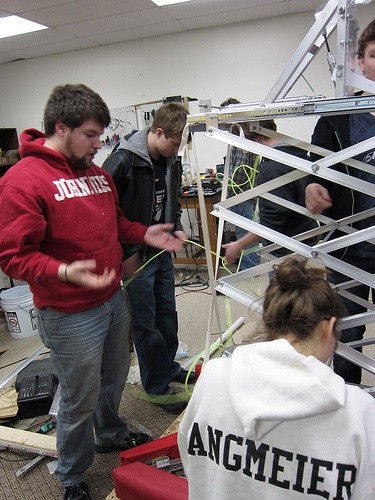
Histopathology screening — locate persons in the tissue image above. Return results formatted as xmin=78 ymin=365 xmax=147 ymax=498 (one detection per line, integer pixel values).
xmin=0 ymin=83 xmax=189 ymax=500
xmin=306 ymin=20 xmax=375 ymax=386
xmin=100 ymin=102 xmax=196 ymax=414
xmin=220 ymin=98 xmax=324 ymax=271
xmin=177 ymin=257 xmax=375 ymax=500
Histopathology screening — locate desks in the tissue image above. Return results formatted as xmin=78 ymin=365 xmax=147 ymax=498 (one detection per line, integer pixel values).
xmin=171 ymin=186 xmax=221 ymax=278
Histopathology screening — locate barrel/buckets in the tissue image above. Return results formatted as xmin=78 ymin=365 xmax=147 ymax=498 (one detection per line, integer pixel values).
xmin=0 ymin=285 xmax=38 ymax=338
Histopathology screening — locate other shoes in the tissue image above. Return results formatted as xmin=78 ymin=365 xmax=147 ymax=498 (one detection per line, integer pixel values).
xmin=61 ymin=481 xmax=89 ymax=500
xmin=149 ymin=387 xmax=187 ymax=413
xmin=171 ymin=368 xmax=197 ymax=384
xmin=95 ymin=431 xmax=153 ymax=453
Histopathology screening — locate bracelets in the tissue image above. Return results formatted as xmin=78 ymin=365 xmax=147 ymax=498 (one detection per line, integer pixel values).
xmin=65 ymin=264 xmax=70 ymax=283
